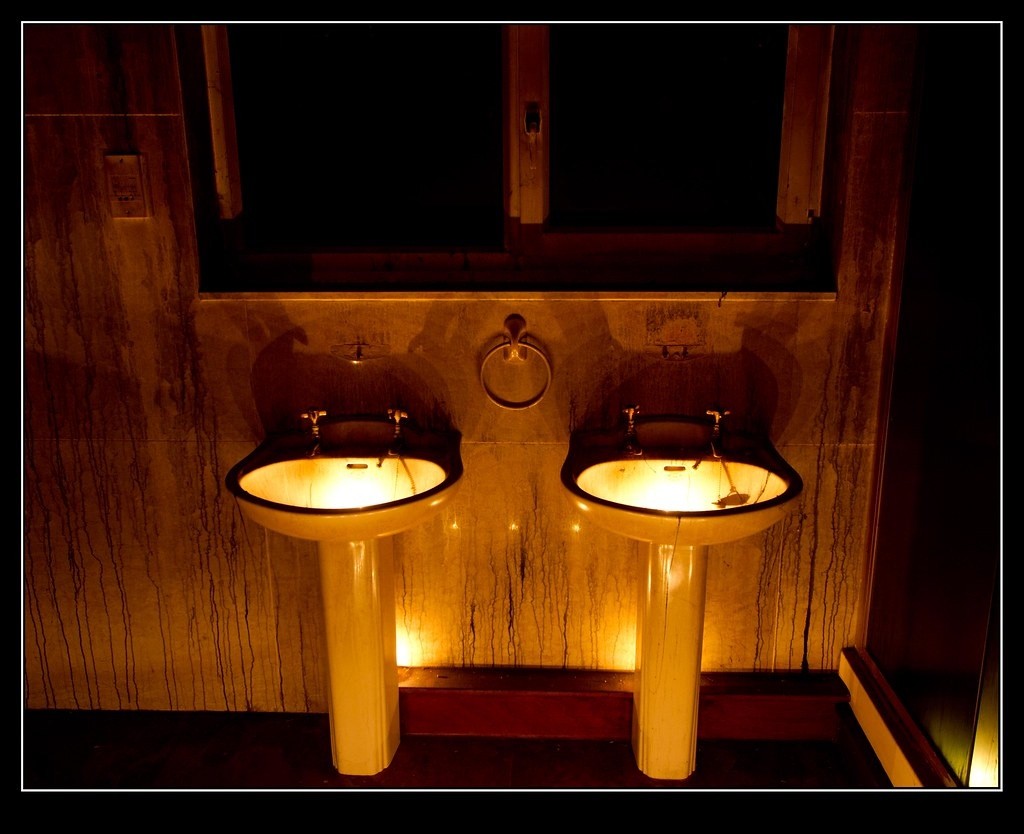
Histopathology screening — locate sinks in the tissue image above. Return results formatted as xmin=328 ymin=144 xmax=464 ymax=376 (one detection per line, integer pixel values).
xmin=557 ymin=430 xmax=805 ymax=548
xmin=221 ymin=412 xmax=465 ymax=544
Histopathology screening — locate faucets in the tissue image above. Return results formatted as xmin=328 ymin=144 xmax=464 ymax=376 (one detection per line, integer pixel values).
xmin=703 ymin=407 xmax=737 ymax=460
xmin=619 ymin=403 xmax=647 ymax=459
xmin=299 ymin=405 xmax=332 ymax=463
xmin=383 ymin=406 xmax=415 ymax=459
